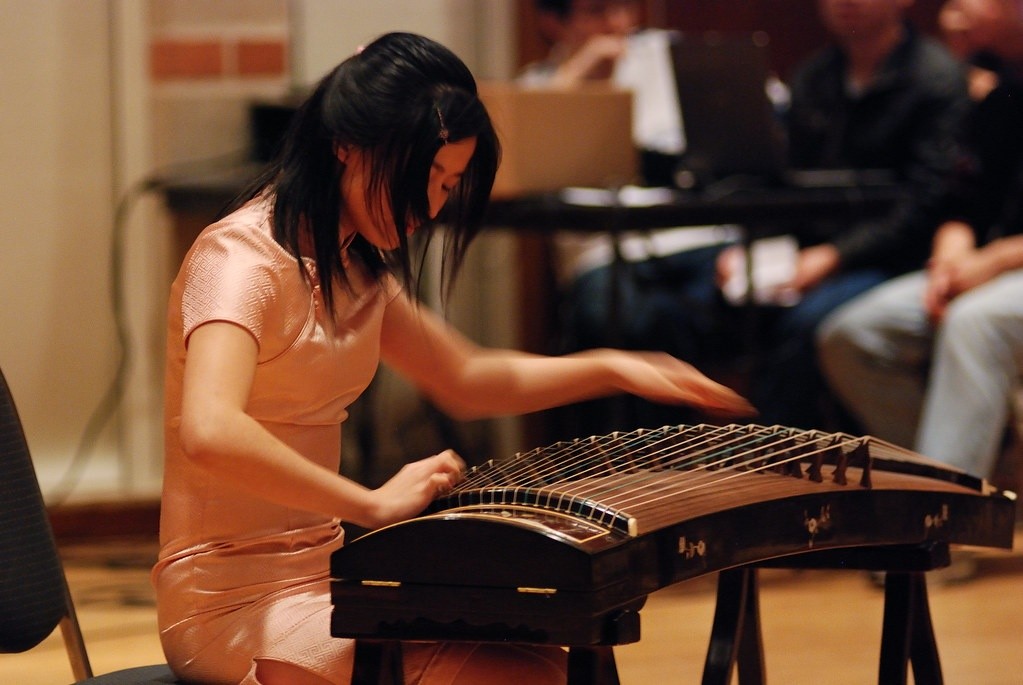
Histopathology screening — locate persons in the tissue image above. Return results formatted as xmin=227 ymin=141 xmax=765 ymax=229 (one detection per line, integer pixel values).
xmin=152 ymin=30 xmax=738 ymax=685
xmin=525 ymin=1 xmax=1023 ymax=590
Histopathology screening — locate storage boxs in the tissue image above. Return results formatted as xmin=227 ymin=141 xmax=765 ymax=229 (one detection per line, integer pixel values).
xmin=470 ymin=85 xmax=639 ymax=200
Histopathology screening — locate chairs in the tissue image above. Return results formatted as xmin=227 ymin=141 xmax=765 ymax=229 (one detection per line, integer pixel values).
xmin=0 ymin=368 xmax=182 ymax=685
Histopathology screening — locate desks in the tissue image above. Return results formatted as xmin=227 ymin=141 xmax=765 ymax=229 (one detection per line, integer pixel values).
xmin=166 ymin=173 xmax=878 ymax=486
